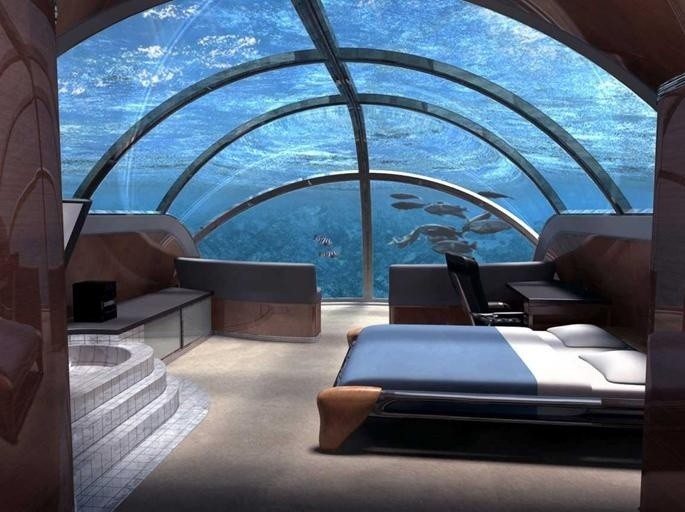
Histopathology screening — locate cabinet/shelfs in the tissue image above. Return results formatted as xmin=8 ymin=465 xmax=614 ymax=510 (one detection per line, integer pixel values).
xmin=67 ymin=285 xmax=215 ymax=362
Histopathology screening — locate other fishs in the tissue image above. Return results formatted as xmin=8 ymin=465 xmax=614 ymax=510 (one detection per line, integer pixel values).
xmin=387 ymin=191 xmax=514 ymax=255
xmin=319 ymin=250 xmax=340 ymax=258
xmin=313 ymin=234 xmax=333 ymax=246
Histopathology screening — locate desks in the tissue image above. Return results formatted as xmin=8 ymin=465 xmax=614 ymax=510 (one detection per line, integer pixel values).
xmin=504 ymin=279 xmax=613 ymax=329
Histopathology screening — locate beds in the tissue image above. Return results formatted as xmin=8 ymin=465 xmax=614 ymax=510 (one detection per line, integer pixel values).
xmin=314 ymin=320 xmax=654 ymax=467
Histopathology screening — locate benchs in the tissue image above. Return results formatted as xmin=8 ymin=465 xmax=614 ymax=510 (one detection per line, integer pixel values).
xmin=175 ymin=256 xmax=323 ymax=342
xmin=386 ymin=261 xmax=548 ymax=324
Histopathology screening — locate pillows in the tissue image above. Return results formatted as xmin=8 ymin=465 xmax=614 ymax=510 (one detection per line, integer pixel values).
xmin=577 ymin=350 xmax=648 ymax=385
xmin=546 ymin=321 xmax=627 ymax=349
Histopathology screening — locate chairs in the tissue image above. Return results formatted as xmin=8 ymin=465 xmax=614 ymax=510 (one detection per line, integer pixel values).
xmin=444 ymin=251 xmax=526 ymax=326
